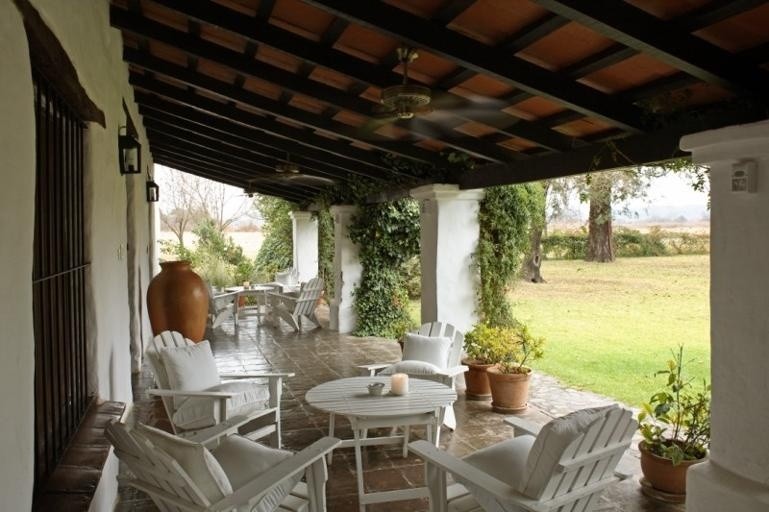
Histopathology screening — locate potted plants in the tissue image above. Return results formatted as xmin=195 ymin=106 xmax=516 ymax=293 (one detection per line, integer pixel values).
xmin=637 ymin=342 xmax=712 ymax=504
xmin=461 ymin=320 xmax=515 ymax=401
xmin=486 ymin=324 xmax=546 ymax=413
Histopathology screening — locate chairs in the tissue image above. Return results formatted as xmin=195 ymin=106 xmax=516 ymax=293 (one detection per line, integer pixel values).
xmin=146 ymin=331 xmax=295 ymax=453
xmin=104 ymin=419 xmax=342 ymax=512
xmin=357 ymin=321 xmax=469 ymax=447
xmin=406 ymin=404 xmax=638 ymax=512
xmin=207 ymin=281 xmax=240 ymax=330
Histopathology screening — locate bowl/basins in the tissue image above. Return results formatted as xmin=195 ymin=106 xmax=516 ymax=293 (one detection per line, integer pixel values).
xmin=367 ymin=383 xmax=385 ymax=396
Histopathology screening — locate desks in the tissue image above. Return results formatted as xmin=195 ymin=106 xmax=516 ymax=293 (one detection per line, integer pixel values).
xmin=305 ymin=374 xmax=457 ymax=512
xmin=224 ymin=285 xmax=274 ymax=329
xmin=268 ymin=278 xmax=325 ymax=333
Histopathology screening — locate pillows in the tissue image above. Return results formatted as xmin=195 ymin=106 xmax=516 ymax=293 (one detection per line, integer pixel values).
xmin=138 ymin=421 xmax=295 ymax=512
xmin=450 ymin=434 xmax=559 ymax=512
xmin=279 ymin=282 xmax=315 ymax=312
xmin=376 ymin=331 xmax=452 ymax=384
xmin=160 ymin=340 xmax=270 ymax=431
